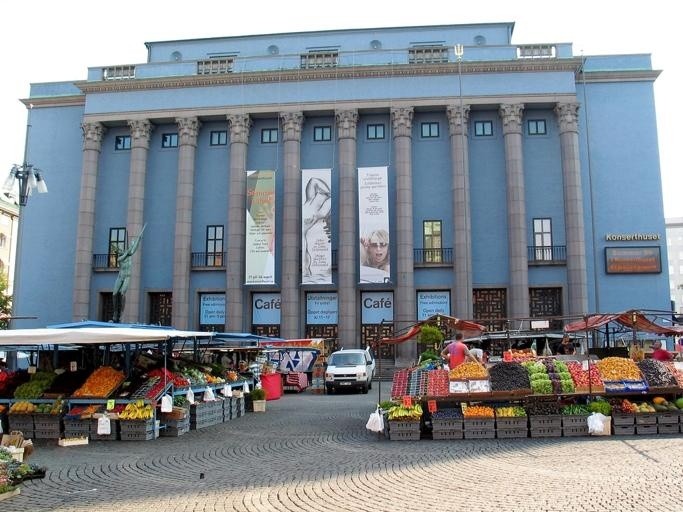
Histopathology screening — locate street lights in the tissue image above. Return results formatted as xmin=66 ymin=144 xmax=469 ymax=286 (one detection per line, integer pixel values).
xmin=4 ymin=163 xmax=48 ymax=328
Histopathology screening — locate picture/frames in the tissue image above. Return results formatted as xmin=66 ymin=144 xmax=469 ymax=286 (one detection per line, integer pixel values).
xmin=604 ymin=246 xmax=663 ymax=275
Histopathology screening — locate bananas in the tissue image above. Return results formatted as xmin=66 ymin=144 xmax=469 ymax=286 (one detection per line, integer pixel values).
xmin=120 ymin=400 xmax=153 ymax=420
xmin=389 ymin=403 xmax=423 ymax=420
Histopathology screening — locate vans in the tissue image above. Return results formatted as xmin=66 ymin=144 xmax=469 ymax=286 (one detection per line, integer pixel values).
xmin=325 ymin=346 xmax=376 ymax=393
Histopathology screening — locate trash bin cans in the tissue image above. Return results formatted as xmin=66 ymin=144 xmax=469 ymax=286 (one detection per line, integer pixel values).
xmin=260 ymin=373 xmax=281 ymax=401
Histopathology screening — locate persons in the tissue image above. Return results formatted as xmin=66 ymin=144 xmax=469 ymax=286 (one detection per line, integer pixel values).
xmin=677 ymin=334 xmax=683 ymax=361
xmin=302 ymin=177 xmax=331 ymax=278
xmin=654 ymin=342 xmax=674 ymax=361
xmin=556 ymin=334 xmax=576 ymax=355
xmin=221 ymin=353 xmax=232 ymax=368
xmin=361 ymin=229 xmax=390 ymax=272
xmin=440 ymin=334 xmax=490 ymax=370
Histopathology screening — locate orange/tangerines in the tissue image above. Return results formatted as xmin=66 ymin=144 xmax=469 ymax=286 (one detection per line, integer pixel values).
xmin=74 ymin=367 xmax=124 ymax=397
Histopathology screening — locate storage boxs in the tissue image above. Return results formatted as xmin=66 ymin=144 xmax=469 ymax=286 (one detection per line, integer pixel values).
xmin=612 ymin=412 xmax=683 ymax=435
xmin=162 ymin=406 xmax=188 ymax=420
